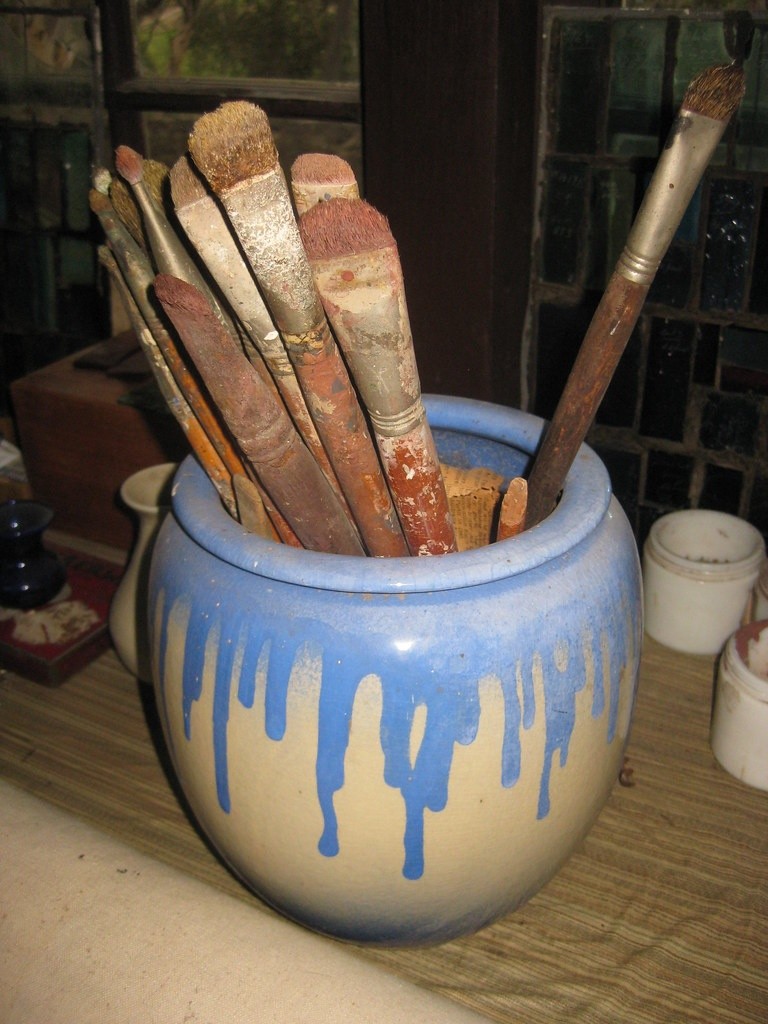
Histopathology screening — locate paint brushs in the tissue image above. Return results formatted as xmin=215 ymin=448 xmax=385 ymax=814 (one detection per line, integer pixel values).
xmin=83 ymin=62 xmax=749 ymax=556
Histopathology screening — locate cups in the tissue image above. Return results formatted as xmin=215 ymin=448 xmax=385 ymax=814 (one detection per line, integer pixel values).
xmin=637 ymin=508 xmax=768 ymax=654
xmin=709 ymin=619 xmax=768 ymax=793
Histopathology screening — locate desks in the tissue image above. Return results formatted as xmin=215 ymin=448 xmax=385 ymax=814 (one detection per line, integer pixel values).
xmin=0 ymin=633 xmax=768 ymax=1024
